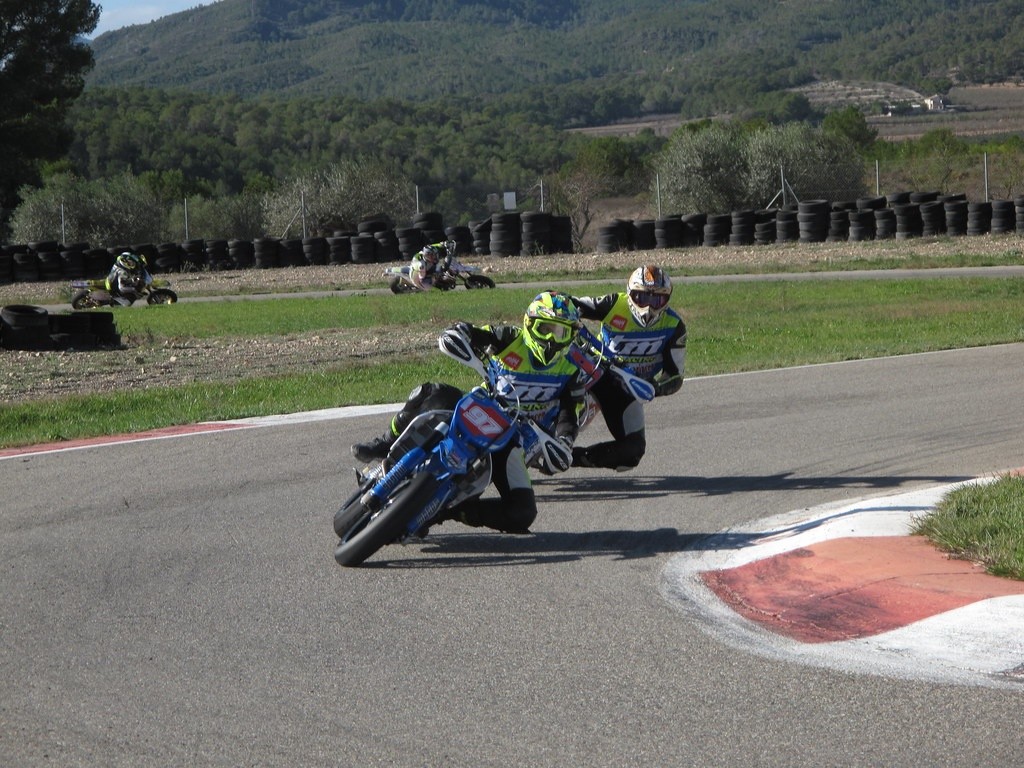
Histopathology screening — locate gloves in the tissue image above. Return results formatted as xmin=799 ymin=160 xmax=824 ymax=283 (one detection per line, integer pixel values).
xmin=447 ymin=321 xmax=474 ymax=344
xmin=644 ymin=376 xmax=662 ymax=397
xmin=556 ymin=435 xmax=573 ymax=454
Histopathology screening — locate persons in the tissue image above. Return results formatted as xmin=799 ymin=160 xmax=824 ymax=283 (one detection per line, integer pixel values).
xmin=556 ymin=264 xmax=686 ymax=473
xmin=409 ymin=240 xmax=452 ymax=294
xmin=349 ymin=291 xmax=588 ymax=531
xmin=105 ymin=252 xmax=146 ymax=306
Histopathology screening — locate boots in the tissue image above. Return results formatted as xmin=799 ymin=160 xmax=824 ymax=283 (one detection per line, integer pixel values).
xmin=419 ymin=499 xmax=482 ymax=539
xmin=351 ymin=412 xmax=406 ymax=463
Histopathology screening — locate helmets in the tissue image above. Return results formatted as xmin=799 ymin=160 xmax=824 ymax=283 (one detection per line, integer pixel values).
xmin=627 ymin=265 xmax=673 ymax=328
xmin=117 ymin=252 xmax=141 ymax=274
xmin=423 ymin=245 xmax=439 ymax=263
xmin=522 ymin=292 xmax=581 ymax=367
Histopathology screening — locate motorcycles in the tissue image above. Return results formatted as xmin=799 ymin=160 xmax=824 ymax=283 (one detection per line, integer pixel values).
xmin=523 ymin=287 xmax=656 ymax=479
xmin=67 ymin=265 xmax=178 ymax=311
xmin=379 ymin=243 xmax=497 ymax=293
xmin=330 ymin=331 xmax=573 ymax=586
